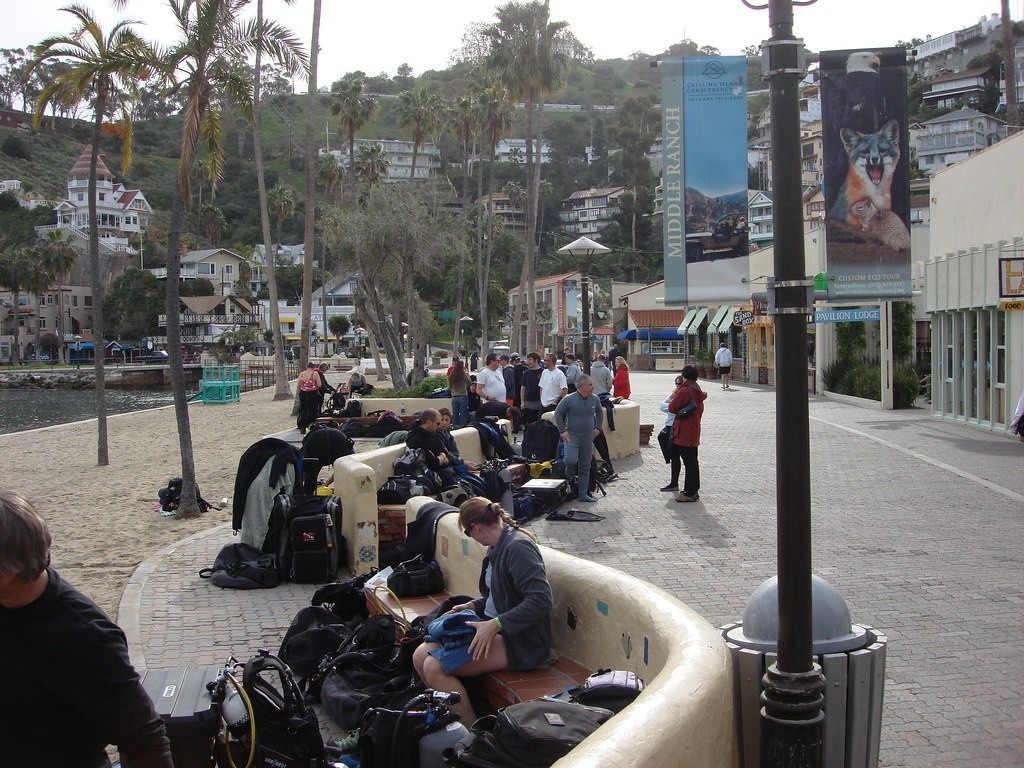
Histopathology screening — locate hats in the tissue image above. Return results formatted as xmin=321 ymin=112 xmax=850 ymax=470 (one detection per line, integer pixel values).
xmin=499 ymin=355 xmax=509 ymax=360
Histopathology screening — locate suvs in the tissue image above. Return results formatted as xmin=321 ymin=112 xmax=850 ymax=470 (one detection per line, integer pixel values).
xmin=685 ymin=213 xmax=748 ymax=262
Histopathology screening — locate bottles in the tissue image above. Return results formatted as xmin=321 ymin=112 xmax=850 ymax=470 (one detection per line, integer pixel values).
xmin=313 ymin=478 xmax=333 ymax=496
xmin=400 ymin=402 xmax=406 ymax=415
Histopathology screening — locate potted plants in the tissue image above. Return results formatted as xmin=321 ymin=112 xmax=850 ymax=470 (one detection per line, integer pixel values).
xmin=704 ymin=350 xmax=718 ymax=379
xmin=694 ymin=349 xmax=704 ymax=377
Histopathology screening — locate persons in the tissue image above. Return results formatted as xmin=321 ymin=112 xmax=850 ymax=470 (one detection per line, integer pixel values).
xmin=298 ymin=362 xmax=322 ymax=433
xmin=590 ymin=350 xmax=609 ymax=368
xmin=519 ymin=352 xmax=546 ymax=424
xmin=1009 ymin=388 xmax=1024 ymax=442
xmin=446 ymin=349 xmax=530 ymax=434
xmin=315 ymin=362 xmax=335 ymax=417
xmin=609 ymin=357 xmax=631 ymax=399
xmin=609 ymin=342 xmax=627 ymax=377
xmin=590 ymin=354 xmax=612 ymax=397
xmin=0 ymin=492 xmax=174 ymax=768
xmin=658 ymin=365 xmax=707 ymax=502
xmin=716 ymin=342 xmax=733 ymax=390
xmin=561 ymin=346 xmax=571 ymax=365
xmin=403 ymin=406 xmax=489 ymax=498
xmin=564 ymin=354 xmax=582 ymax=394
xmin=720 ymin=215 xmax=746 ymax=232
xmin=538 ymin=353 xmax=567 ymax=419
xmin=347 ymin=371 xmax=366 ymax=398
xmin=412 ymin=498 xmax=555 ymax=735
xmin=554 ymin=373 xmax=603 ymax=501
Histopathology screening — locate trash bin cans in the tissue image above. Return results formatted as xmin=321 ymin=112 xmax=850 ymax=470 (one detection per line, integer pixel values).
xmin=477 ymin=359 xmax=483 ymax=369
xmin=718 ymin=576 xmax=887 ymax=768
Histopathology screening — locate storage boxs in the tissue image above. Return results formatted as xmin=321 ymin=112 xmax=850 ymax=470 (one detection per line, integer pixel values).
xmin=521 ymin=479 xmax=568 ymax=511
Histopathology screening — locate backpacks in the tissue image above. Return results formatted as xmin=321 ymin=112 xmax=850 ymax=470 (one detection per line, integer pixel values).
xmin=158 ymin=393 xmax=646 ymax=768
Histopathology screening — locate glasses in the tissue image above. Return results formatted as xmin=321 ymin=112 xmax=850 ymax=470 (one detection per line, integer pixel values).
xmin=676 ymin=382 xmax=683 ymax=385
xmin=544 ymin=359 xmax=552 ymax=362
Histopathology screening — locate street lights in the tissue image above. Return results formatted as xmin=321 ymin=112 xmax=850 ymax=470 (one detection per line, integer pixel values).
xmin=555 ymin=236 xmax=612 ymax=374
xmin=459 ymin=316 xmax=474 ymax=374
xmin=356 ymin=326 xmax=364 ymax=365
xmin=10 ymin=337 xmax=14 ymax=366
xmin=73 ymin=334 xmax=82 ymax=369
xmin=497 ymin=319 xmax=503 ymax=339
xmin=312 ymin=328 xmax=319 ymax=357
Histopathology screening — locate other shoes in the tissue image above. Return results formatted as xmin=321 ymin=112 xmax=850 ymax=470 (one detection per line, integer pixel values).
xmin=726 ymin=385 xmax=729 ymax=388
xmin=661 ymin=485 xmax=679 ymax=491
xmin=579 ymin=494 xmax=595 ymax=502
xmin=721 ymin=386 xmax=725 ymax=389
xmin=676 ymin=491 xmax=699 ymax=502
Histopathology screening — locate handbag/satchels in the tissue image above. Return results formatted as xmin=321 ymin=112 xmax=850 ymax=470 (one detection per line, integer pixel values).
xmin=676 ymin=388 xmax=697 ymax=417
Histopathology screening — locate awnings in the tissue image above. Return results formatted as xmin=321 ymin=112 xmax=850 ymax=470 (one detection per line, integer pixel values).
xmin=286 ymin=335 xmax=301 ymax=341
xmin=639 ymin=328 xmax=684 ymax=340
xmin=279 ymin=318 xmax=296 ymax=323
xmin=319 ymin=335 xmax=343 ymax=342
xmin=616 ymin=329 xmax=637 ymax=340
xmin=719 ymin=306 xmax=741 ymax=334
xmin=676 ymin=309 xmax=696 ymax=335
xmin=688 ymin=307 xmax=707 ymax=336
xmin=706 ymin=305 xmax=727 ymax=335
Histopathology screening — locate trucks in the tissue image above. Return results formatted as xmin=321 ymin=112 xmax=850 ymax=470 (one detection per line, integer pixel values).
xmin=136 ymin=350 xmax=168 ymax=364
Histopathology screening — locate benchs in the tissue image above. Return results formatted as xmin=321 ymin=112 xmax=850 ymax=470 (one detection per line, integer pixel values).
xmin=317 ymin=398 xmax=453 ymax=428
xmin=334 ymin=419 xmax=512 ymax=577
xmin=365 ymin=496 xmax=739 ymax=768
xmin=542 ymin=397 xmax=640 ymax=462
